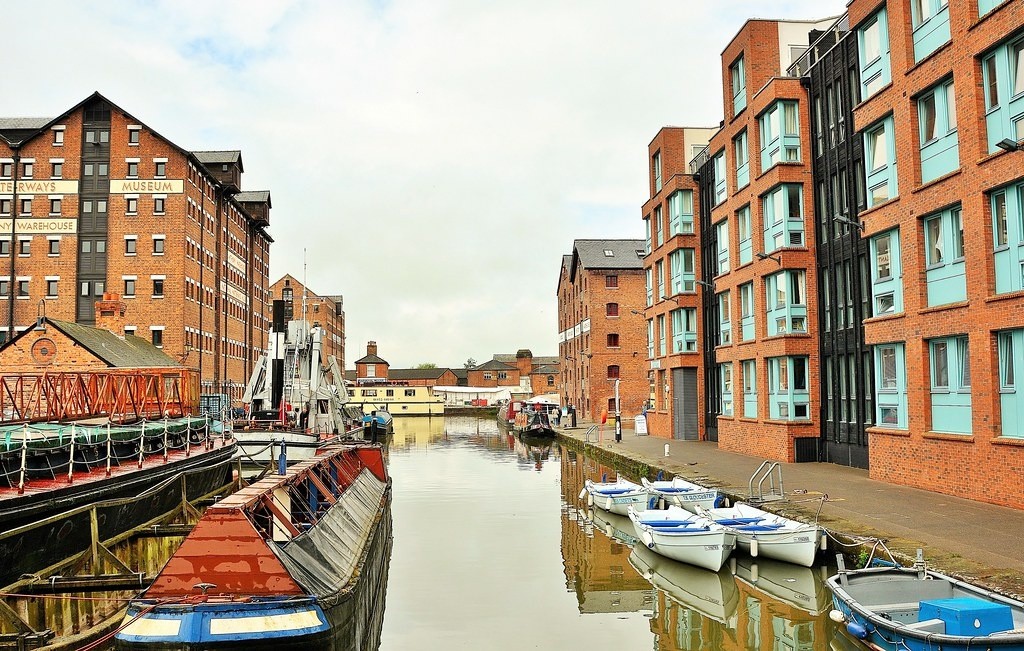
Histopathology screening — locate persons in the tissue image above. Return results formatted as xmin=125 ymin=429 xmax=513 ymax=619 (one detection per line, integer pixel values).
xmin=552 ymin=407 xmax=562 ymax=426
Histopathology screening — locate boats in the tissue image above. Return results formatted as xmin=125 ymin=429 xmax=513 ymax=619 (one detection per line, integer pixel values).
xmin=0 ymin=367 xmax=239 ymax=590
xmin=627 ymin=504 xmax=738 ymax=572
xmin=826 ymin=548 xmax=1024 ymax=651
xmin=117 ymin=443 xmax=392 ymax=651
xmin=514 ymin=436 xmax=554 ymax=473
xmin=640 ymin=476 xmax=718 ymax=515
xmin=584 ymin=473 xmax=662 ymax=517
xmin=587 ymin=504 xmax=641 ymax=545
xmin=496 ymin=400 xmax=526 ymax=427
xmin=513 ymin=408 xmax=556 ymax=441
xmin=345 ymin=385 xmax=445 ymax=417
xmin=627 ymin=540 xmax=740 ymax=625
xmin=694 ymin=501 xmax=824 ymax=567
xmin=232 ymin=409 xmax=319 ymax=465
xmin=358 ymin=411 xmax=393 ymax=435
xmin=724 ymin=550 xmax=838 ymax=617
